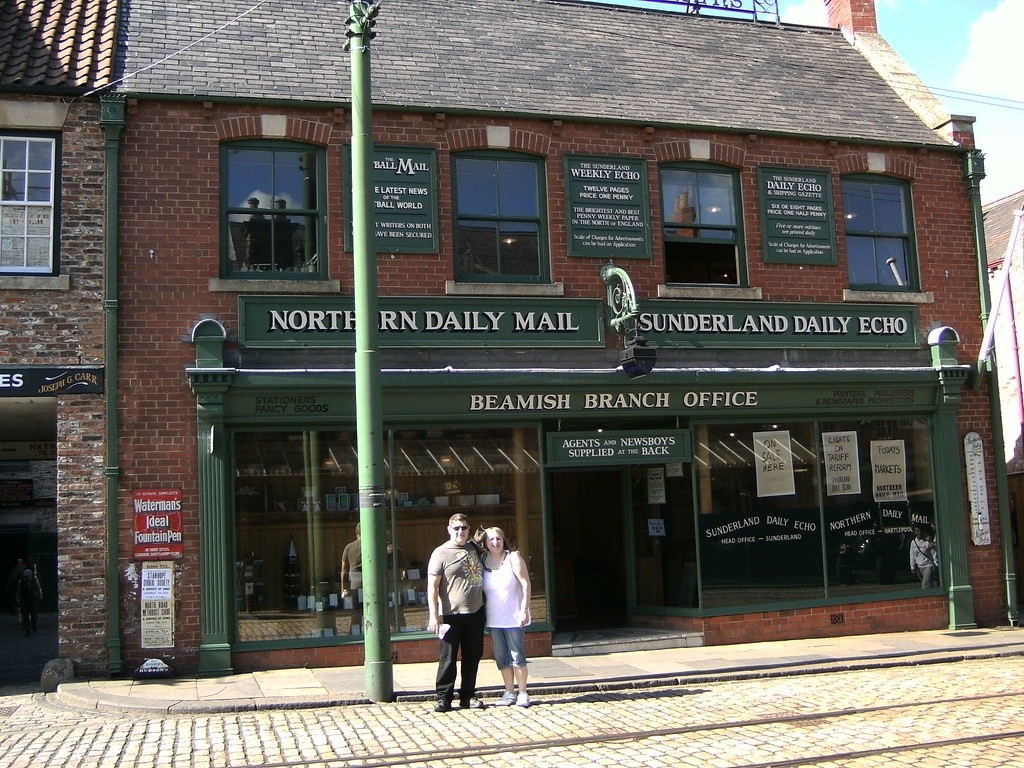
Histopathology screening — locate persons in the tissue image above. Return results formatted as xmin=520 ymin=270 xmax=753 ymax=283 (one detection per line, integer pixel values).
xmin=929 ymin=519 xmax=940 ymax=567
xmin=473 ymin=525 xmax=532 ymax=707
xmin=339 ymin=522 xmax=364 ymax=635
xmin=427 ymin=513 xmax=487 ymax=710
xmin=909 ymin=525 xmax=936 ymax=590
xmin=386 ymin=530 xmax=407 ymax=631
xmin=15 ymin=569 xmax=43 ymax=636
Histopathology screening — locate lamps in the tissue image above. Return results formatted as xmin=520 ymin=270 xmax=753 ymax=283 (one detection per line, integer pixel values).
xmin=618 ymin=316 xmax=660 ymax=381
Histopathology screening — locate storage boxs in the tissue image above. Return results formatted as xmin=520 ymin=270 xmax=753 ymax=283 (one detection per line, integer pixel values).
xmin=432 ymin=496 xmax=449 ymax=506
xmin=475 ymin=494 xmax=500 ymax=505
xmin=450 ymin=495 xmax=475 ymax=506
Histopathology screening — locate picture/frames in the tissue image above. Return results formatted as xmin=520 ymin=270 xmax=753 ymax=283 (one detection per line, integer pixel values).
xmin=323 ymin=487 xmax=413 ymax=511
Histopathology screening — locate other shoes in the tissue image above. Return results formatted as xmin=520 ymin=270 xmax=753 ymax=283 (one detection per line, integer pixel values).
xmin=501 ymin=691 xmax=517 ymax=706
xmin=434 ymin=700 xmax=451 ymax=712
xmin=459 ymin=697 xmax=483 ymax=707
xmin=516 ymin=691 xmax=530 ymax=706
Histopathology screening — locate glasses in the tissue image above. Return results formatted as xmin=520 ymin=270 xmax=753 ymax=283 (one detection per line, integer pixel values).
xmin=449 ymin=525 xmax=469 ymax=530
